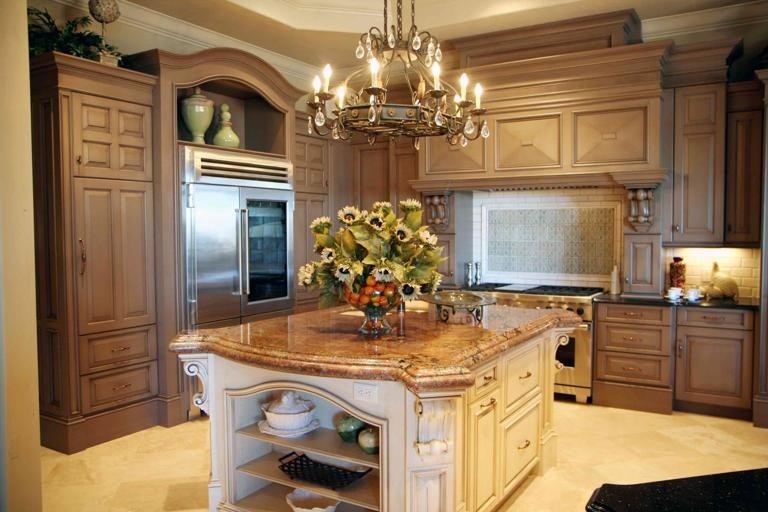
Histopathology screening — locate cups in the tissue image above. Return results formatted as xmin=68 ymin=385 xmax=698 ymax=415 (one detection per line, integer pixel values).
xmin=666 ymin=286 xmax=683 ymax=300
xmin=686 ymin=289 xmax=699 ymax=302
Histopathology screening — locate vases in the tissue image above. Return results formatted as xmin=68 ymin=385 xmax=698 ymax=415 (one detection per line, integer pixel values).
xmin=181 ymin=83 xmax=216 ymax=145
xmin=344 ymin=280 xmax=401 ymax=336
xmin=669 ymin=255 xmax=686 ymax=293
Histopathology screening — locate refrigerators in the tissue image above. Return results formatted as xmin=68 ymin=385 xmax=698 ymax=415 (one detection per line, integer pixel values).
xmin=178 ymin=181 xmax=297 ymax=423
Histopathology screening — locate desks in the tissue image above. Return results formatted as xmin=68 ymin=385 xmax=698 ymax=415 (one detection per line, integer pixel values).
xmin=584 ymin=466 xmax=768 ymax=511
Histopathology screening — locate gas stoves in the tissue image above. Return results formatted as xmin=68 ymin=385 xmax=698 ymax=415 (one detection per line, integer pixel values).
xmin=519 ymin=285 xmax=603 ymax=298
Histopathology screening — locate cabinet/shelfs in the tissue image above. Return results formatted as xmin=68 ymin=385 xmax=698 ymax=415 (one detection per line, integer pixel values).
xmin=409 ymin=38 xmax=672 ymax=194
xmin=674 ymin=302 xmax=760 ymax=422
xmin=31 ymin=174 xmax=154 ymax=335
xmin=501 ymin=337 xmax=540 ymax=501
xmin=727 ymin=78 xmax=765 ymax=249
xmin=593 ymin=299 xmax=676 ymax=416
xmin=622 ymin=230 xmax=666 ymax=298
xmin=222 ymin=380 xmax=390 ymax=512
xmin=466 ymin=354 xmax=501 ymax=511
xmin=29 ymin=51 xmax=159 ymax=179
xmin=421 ymin=191 xmax=472 ymax=286
xmin=290 ymin=79 xmax=421 ymax=310
xmin=664 ymin=37 xmax=747 ymax=248
xmin=36 ymin=323 xmax=157 ymax=457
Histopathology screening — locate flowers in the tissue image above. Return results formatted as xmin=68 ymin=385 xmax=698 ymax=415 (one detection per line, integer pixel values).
xmin=297 ymin=197 xmax=448 ymax=301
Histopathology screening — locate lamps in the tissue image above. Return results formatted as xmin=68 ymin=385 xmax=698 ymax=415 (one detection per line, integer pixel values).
xmin=307 ymin=1 xmax=491 ymax=153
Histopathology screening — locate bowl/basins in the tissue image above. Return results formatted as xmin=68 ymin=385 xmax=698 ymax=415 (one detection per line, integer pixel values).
xmin=285 ymin=488 xmax=342 ymax=512
xmin=260 ymin=389 xmax=317 ymax=431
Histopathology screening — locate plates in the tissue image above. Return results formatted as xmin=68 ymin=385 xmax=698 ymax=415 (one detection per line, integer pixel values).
xmin=257 ymin=417 xmax=320 ymax=437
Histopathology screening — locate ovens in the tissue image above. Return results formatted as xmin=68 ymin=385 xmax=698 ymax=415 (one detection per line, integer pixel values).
xmin=553 ymin=320 xmax=593 ymax=405
